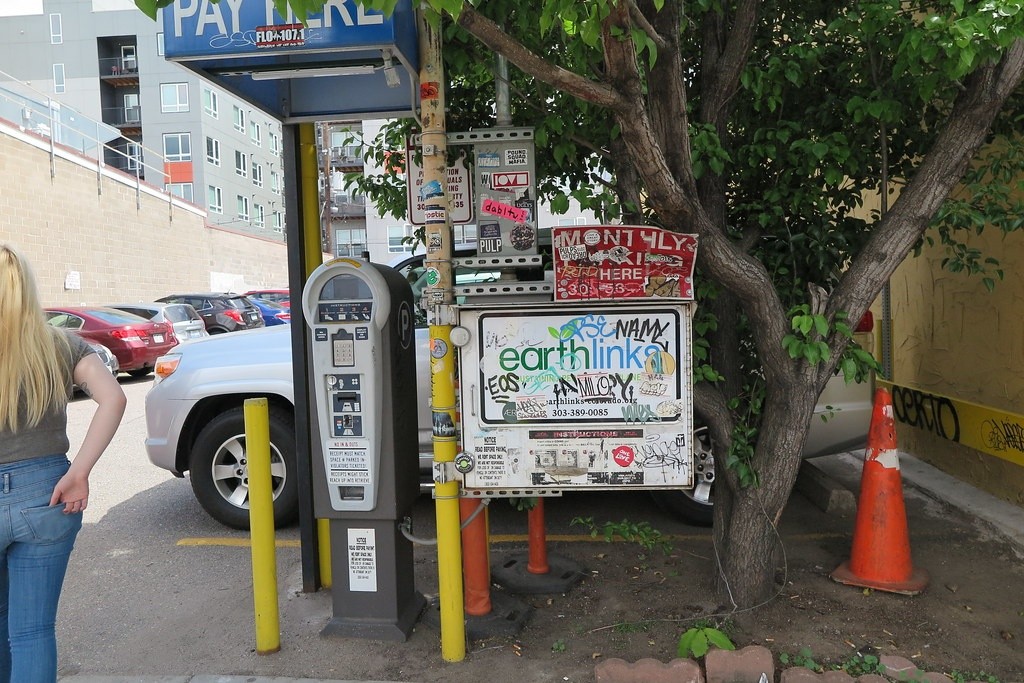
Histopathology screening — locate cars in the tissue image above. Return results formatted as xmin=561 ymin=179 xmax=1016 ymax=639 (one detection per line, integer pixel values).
xmin=146 ymin=229 xmax=876 ymax=533
xmin=250 ymin=297 xmax=291 ymax=327
xmin=42 ymin=305 xmax=180 ymax=379
xmin=72 ymin=337 xmax=120 ymax=392
xmin=54 ymin=304 xmax=211 ymax=345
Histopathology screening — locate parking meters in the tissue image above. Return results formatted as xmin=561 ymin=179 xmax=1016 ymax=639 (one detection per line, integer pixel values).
xmin=302 ymin=258 xmax=429 ymax=643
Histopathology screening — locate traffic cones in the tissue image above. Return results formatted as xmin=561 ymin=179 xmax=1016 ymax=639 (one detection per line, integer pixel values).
xmin=826 ymin=385 xmax=929 ymax=597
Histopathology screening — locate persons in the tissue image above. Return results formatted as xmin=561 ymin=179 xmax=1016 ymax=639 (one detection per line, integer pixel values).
xmin=0 ymin=242 xmax=127 ymax=683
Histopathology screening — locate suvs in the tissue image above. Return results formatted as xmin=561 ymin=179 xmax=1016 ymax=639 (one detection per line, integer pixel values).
xmin=244 ymin=289 xmax=290 ymax=309
xmin=153 ymin=292 xmax=266 ymax=336
